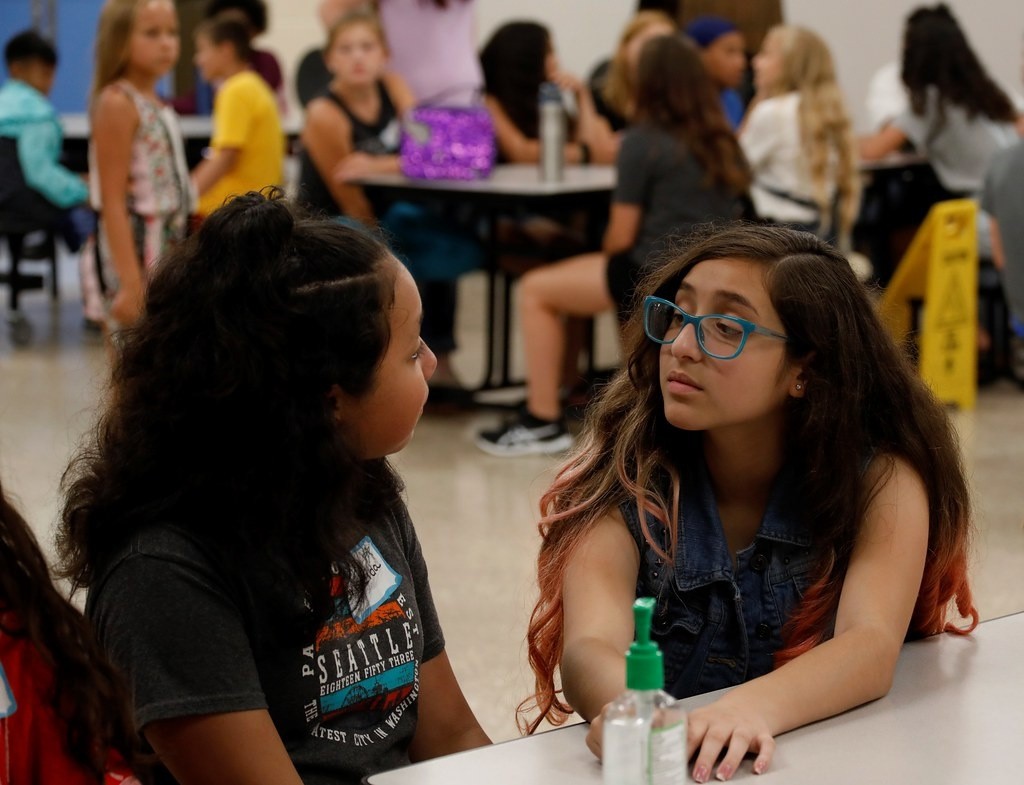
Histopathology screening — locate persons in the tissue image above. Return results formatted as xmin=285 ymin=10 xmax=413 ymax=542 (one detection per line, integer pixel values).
xmin=516 ymin=224 xmax=978 ymax=785
xmin=0 ymin=2 xmax=1021 ymax=457
xmin=0 ymin=484 xmax=160 ymax=785
xmin=48 ymin=191 xmax=495 ymax=785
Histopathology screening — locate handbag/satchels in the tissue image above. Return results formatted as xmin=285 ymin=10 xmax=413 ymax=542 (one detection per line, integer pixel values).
xmin=401 ymin=104 xmax=498 ymax=184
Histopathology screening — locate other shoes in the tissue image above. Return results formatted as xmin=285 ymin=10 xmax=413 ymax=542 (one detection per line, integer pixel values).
xmin=511 ymin=379 xmax=595 ymax=421
xmin=83 ymin=315 xmax=105 ymax=330
xmin=473 ymin=411 xmax=574 ymax=457
xmin=434 ymin=380 xmax=474 ymax=404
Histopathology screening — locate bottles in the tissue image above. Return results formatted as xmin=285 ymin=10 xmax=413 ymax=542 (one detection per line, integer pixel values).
xmin=539 ymin=84 xmax=563 ymax=184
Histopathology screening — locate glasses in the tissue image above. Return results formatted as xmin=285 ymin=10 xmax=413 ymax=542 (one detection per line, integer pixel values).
xmin=641 ymin=293 xmax=789 ymax=360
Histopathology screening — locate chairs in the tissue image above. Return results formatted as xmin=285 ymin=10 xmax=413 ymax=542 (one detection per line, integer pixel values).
xmin=0 ymin=135 xmax=58 ymax=310
xmin=977 ymin=143 xmax=1024 ymax=387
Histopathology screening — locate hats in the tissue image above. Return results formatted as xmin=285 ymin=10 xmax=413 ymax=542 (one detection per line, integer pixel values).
xmin=685 ymin=18 xmax=740 ymax=48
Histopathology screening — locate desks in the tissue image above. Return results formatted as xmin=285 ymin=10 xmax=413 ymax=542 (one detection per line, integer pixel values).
xmin=361 ymin=612 xmax=1024 ymax=784
xmin=61 ymin=115 xmax=298 ymax=139
xmin=347 ymin=152 xmax=915 ymax=407
